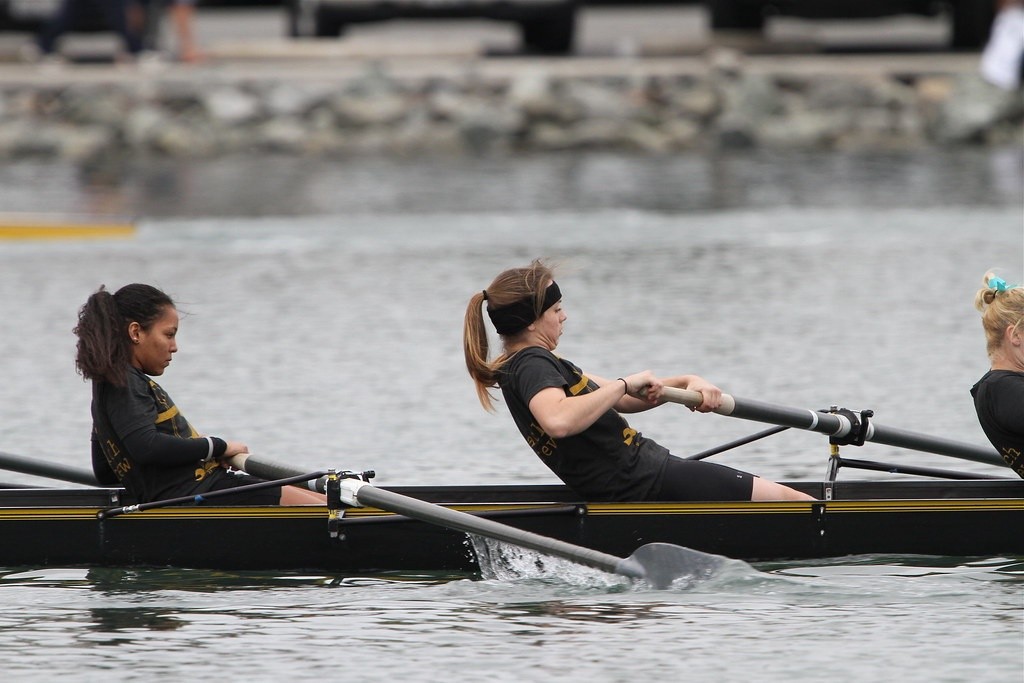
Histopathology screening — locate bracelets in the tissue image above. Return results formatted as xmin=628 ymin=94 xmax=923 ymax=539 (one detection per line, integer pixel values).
xmin=618 ymin=377 xmax=627 ymax=394
xmin=201 ymin=436 xmax=214 ymax=462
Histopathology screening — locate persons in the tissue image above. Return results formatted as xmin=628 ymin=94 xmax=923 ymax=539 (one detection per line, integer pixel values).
xmin=74 ymin=283 xmax=329 ymax=505
xmin=463 ymin=264 xmax=821 ymax=502
xmin=971 ymin=271 xmax=1024 ymax=478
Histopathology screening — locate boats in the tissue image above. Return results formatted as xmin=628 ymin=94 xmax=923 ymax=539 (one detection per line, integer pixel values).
xmin=0 ymin=477 xmax=1024 ymax=575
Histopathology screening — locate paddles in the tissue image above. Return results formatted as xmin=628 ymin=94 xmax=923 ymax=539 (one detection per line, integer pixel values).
xmin=637 ymin=383 xmax=1013 ymax=468
xmin=222 ymin=453 xmax=746 ymax=587
xmin=0 ymin=455 xmax=110 ymax=488
